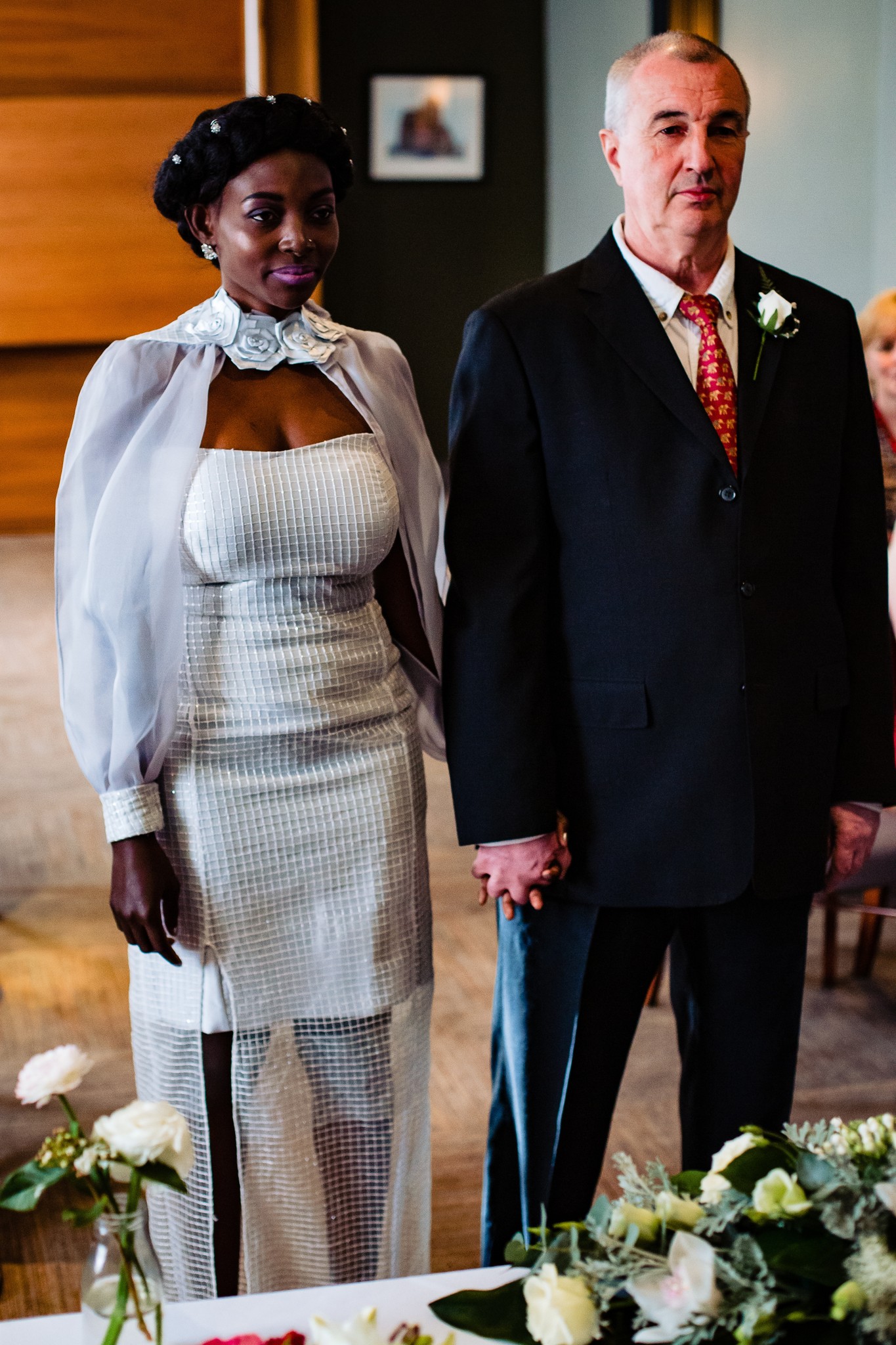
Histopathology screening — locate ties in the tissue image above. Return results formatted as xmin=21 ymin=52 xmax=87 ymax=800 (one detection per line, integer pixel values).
xmin=676 ymin=293 xmax=740 ymax=470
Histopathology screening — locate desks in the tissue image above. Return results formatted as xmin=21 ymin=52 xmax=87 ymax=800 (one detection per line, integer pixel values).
xmin=1 ymin=1263 xmax=538 ymax=1345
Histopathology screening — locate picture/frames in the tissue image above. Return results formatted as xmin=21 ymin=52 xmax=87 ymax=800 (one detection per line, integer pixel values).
xmin=369 ymin=75 xmax=486 ymax=182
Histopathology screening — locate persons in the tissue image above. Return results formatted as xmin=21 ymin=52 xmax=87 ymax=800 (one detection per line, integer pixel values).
xmin=852 ymin=284 xmax=896 ymax=564
xmin=49 ymin=94 xmax=569 ymax=1305
xmin=432 ymin=34 xmax=894 ymax=1273
